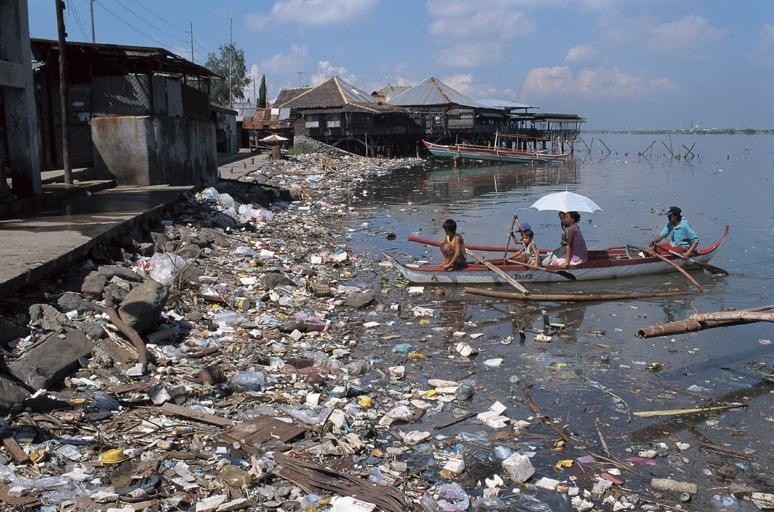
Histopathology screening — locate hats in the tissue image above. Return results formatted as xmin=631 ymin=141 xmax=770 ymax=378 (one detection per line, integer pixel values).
xmin=663 ymin=208 xmax=681 ymax=217
xmin=515 ymin=224 xmax=530 ymax=232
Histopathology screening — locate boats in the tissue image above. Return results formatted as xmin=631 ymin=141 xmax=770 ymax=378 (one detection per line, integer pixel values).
xmin=386 ymin=225 xmax=733 ymax=284
xmin=634 ymin=306 xmax=774 ymax=340
xmin=421 ymin=137 xmax=570 ymax=164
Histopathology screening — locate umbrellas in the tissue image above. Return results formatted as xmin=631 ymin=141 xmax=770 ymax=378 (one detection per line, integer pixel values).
xmin=528 ymin=186 xmax=604 ymax=245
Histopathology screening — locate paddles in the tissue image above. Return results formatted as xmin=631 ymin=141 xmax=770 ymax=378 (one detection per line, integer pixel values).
xmin=507 ymin=259 xmax=576 ymax=281
xmin=650 ymin=242 xmax=729 ymax=276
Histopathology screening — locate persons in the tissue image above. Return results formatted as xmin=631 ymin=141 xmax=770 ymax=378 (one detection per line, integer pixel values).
xmin=540 ymin=212 xmax=572 ymax=268
xmin=503 ymin=230 xmax=541 ymax=270
xmin=439 ymin=218 xmax=468 ymax=270
xmin=511 ymin=222 xmax=531 ymax=246
xmin=628 ymin=207 xmax=699 ymax=259
xmin=550 ymin=211 xmax=588 ymax=268
